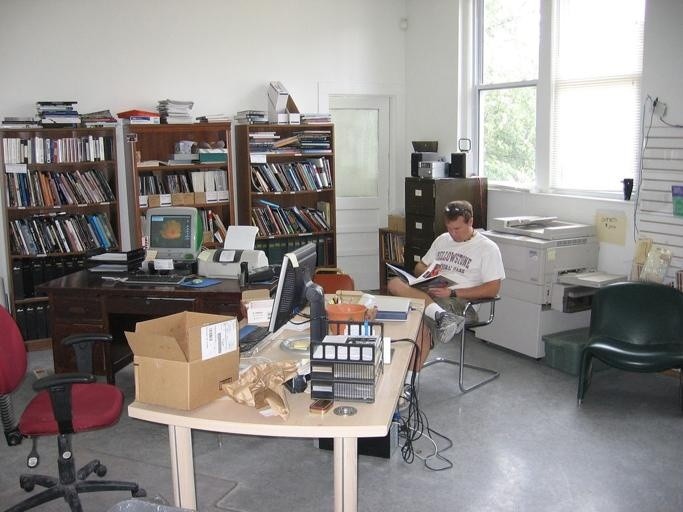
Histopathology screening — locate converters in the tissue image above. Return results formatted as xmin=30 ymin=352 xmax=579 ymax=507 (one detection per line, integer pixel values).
xmin=652 ymin=98 xmax=658 ymax=107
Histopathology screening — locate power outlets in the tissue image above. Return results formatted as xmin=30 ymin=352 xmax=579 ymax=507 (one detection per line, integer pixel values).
xmin=649 ymin=101 xmax=666 ymax=117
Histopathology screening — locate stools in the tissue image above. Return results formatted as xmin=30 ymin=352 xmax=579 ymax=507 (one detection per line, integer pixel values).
xmin=577 ymin=282 xmax=683 ymax=405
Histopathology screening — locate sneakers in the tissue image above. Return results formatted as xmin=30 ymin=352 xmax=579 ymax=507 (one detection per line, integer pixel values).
xmin=395 ymin=384 xmax=417 ymax=414
xmin=434 ymin=311 xmax=466 ymax=344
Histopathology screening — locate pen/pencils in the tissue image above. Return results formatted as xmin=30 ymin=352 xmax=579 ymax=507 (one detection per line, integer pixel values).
xmin=332 ymin=293 xmax=353 ymax=304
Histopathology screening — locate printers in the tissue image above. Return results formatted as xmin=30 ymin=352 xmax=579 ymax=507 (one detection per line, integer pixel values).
xmin=198 ymin=225 xmax=269 ymax=279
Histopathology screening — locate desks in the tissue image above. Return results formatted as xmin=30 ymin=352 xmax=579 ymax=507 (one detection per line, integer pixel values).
xmin=34 ymin=266 xmax=279 ymax=386
xmin=127 ymin=294 xmax=425 ymax=512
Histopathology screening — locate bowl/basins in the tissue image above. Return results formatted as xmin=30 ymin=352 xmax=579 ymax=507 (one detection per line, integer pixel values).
xmin=327 ymin=303 xmax=369 ymax=337
xmin=335 ymin=289 xmax=363 ymax=304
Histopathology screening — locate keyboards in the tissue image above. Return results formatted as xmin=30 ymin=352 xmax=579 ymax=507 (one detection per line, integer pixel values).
xmin=240 ymin=324 xmax=271 ymax=352
xmin=124 ymin=273 xmax=187 ymax=284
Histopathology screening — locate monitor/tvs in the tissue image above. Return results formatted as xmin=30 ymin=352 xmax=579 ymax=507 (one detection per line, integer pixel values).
xmin=143 ymin=207 xmax=203 ymax=261
xmin=268 ymin=243 xmax=329 ymax=345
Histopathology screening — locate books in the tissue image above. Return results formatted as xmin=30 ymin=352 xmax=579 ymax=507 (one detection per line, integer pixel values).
xmin=157 ymin=101 xmax=193 ymax=124
xmin=196 ymin=115 xmax=231 ymax=123
xmin=90 ymin=246 xmax=144 ymax=273
xmin=138 ymin=170 xmax=229 ymax=207
xmin=386 ymin=261 xmax=458 ymax=291
xmin=251 ymin=157 xmax=332 ymax=192
xmin=200 ymin=210 xmax=227 ymax=244
xmin=249 ymin=130 xmax=333 ymax=154
xmin=118 ymin=110 xmax=160 ymax=124
xmin=235 ymin=110 xmax=267 ymax=124
xmin=10 ymin=212 xmax=118 ymax=255
xmin=251 ymin=200 xmax=330 ymax=236
xmin=2 ymin=135 xmax=105 ymax=163
xmin=137 ymin=140 xmax=228 ymax=167
xmin=379 ymin=231 xmax=406 ymax=267
xmin=0 ymin=101 xmax=118 ymax=129
xmin=9 ymin=168 xmax=116 ymax=206
xmin=301 ymin=112 xmax=332 ymax=124
xmin=370 ymin=297 xmax=410 ymax=322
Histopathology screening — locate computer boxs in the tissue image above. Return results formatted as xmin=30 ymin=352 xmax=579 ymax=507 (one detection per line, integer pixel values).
xmin=314 ymin=411 xmax=400 ymax=459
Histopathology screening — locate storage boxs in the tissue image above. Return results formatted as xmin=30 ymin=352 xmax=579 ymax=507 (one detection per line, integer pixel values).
xmin=388 ymin=215 xmax=406 ymax=233
xmin=124 ymin=311 xmax=239 ymax=410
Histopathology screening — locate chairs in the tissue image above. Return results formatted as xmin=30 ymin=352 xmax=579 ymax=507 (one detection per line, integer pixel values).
xmin=313 ymin=268 xmax=354 ymax=294
xmin=422 ymin=296 xmax=500 ymax=393
xmin=0 ymin=304 xmax=147 ymax=512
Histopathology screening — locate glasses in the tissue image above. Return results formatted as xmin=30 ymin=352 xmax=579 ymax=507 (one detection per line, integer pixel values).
xmin=443 ymin=206 xmax=466 ymax=216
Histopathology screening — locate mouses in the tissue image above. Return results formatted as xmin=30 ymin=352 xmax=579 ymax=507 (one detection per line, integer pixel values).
xmin=193 ymin=280 xmax=205 ymax=287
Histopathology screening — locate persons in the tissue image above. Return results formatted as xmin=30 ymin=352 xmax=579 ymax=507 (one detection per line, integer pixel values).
xmin=386 ymin=199 xmax=506 ymax=411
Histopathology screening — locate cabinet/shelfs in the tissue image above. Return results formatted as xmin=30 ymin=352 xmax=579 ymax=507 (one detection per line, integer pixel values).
xmin=0 ymin=120 xmax=122 ymax=343
xmin=123 ymin=121 xmax=235 ymax=252
xmin=235 ymin=124 xmax=337 ymax=274
xmin=405 ymin=176 xmax=487 ymax=277
xmin=379 ymin=228 xmax=406 ymax=295
